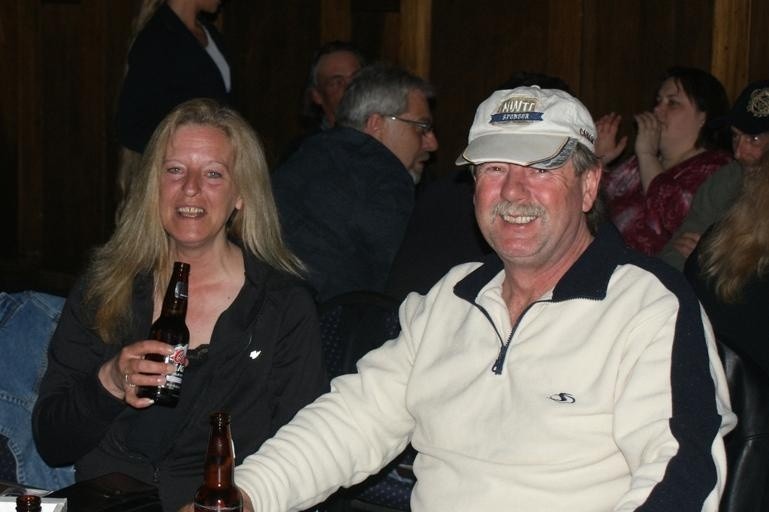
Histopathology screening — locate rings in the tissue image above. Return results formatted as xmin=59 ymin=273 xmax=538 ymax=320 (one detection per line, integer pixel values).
xmin=123 ymin=374 xmax=132 ymax=386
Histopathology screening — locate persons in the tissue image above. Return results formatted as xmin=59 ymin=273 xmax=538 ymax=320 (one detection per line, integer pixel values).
xmin=277 ymin=40 xmax=365 ymax=168
xmin=385 ymin=73 xmax=650 ymax=304
xmin=180 ymin=83 xmax=739 ymax=511
xmin=682 ymin=141 xmax=768 ymax=383
xmin=118 ymin=1 xmax=240 ymax=202
xmin=267 ymin=63 xmax=438 ymax=307
xmin=660 ymin=79 xmax=768 ymax=274
xmin=592 ymin=64 xmax=734 ymax=256
xmin=30 ymin=93 xmax=330 ymax=512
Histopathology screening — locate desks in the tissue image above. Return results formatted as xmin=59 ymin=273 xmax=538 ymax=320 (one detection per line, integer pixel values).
xmin=0 ymin=483 xmax=396 ymax=512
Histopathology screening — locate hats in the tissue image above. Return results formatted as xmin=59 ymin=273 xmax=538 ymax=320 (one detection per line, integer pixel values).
xmin=729 ymin=84 xmax=768 ymax=135
xmin=454 ymin=84 xmax=598 ymax=171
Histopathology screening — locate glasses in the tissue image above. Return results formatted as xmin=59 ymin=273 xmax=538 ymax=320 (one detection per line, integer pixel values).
xmin=392 ymin=115 xmax=431 ymax=138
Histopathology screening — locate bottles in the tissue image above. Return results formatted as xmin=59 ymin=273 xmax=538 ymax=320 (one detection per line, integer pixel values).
xmin=16 ymin=495 xmax=42 ymax=512
xmin=138 ymin=261 xmax=190 ymax=408
xmin=194 ymin=410 xmax=244 ymax=512
